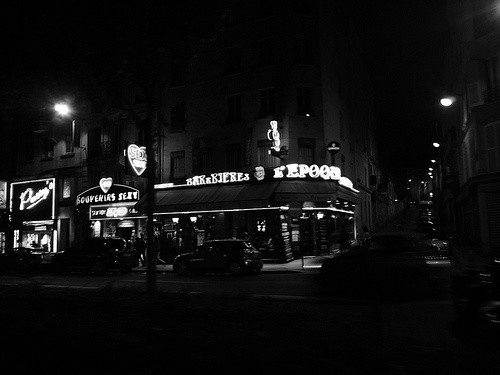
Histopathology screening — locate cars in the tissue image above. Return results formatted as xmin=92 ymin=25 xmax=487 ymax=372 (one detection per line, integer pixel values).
xmin=414 ymin=195 xmax=436 ymax=232
xmin=1 ymin=246 xmax=53 ymax=275
xmin=172 ymin=239 xmax=263 ymax=277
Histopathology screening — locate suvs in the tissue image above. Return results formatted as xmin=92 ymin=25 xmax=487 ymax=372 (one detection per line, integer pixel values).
xmin=50 ymin=237 xmax=141 ymax=277
